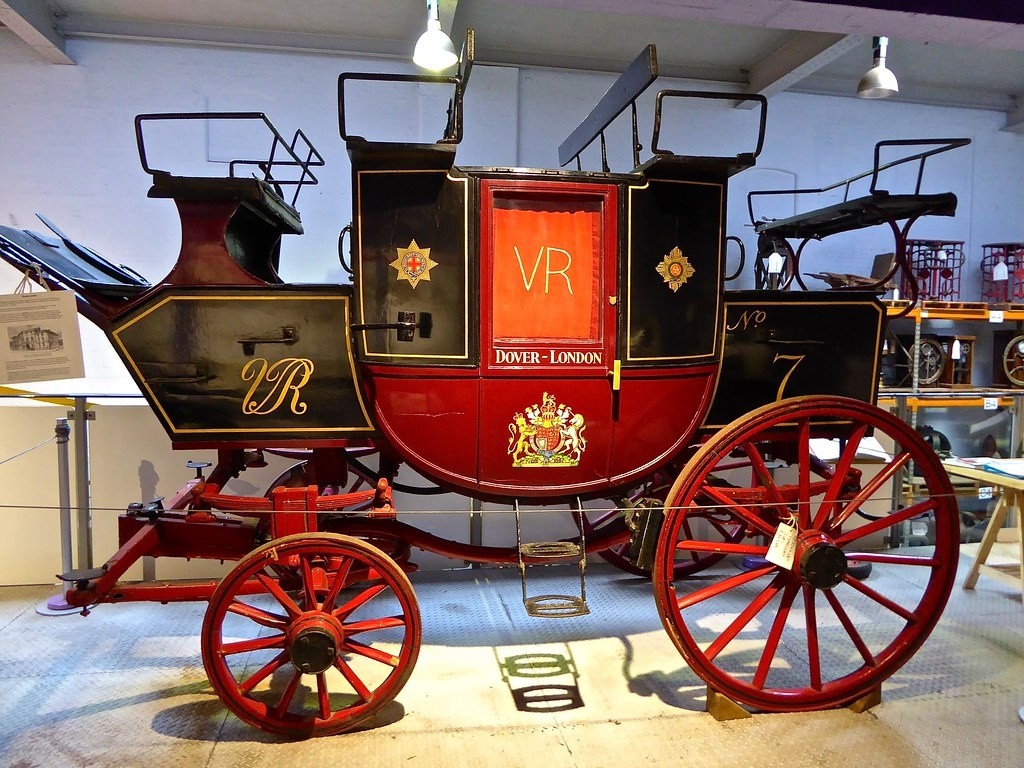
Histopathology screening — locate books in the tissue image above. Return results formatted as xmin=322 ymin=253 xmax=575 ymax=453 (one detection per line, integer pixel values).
xmin=808 ymin=436 xmax=886 ymax=463
xmin=944 ymin=452 xmax=994 ymax=469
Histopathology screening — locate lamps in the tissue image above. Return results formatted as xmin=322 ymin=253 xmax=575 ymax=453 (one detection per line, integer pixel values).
xmin=857 ymin=36 xmax=899 ymax=99
xmin=413 ymin=0 xmax=458 ymax=71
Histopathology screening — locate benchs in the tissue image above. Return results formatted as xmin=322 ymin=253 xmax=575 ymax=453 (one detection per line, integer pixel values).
xmin=557 ymin=44 xmax=767 ymax=178
xmin=135 ymin=112 xmax=325 ymax=283
xmin=747 ymin=138 xmax=971 ymax=319
xmin=337 ymin=28 xmax=474 ymax=164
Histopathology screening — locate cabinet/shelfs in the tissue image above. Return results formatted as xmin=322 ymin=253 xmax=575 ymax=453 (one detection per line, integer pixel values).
xmin=879 ymin=299 xmax=1024 ymax=549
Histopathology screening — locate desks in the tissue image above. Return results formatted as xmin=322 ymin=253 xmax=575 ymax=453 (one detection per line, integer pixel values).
xmin=940 ymin=458 xmax=1024 ymax=593
